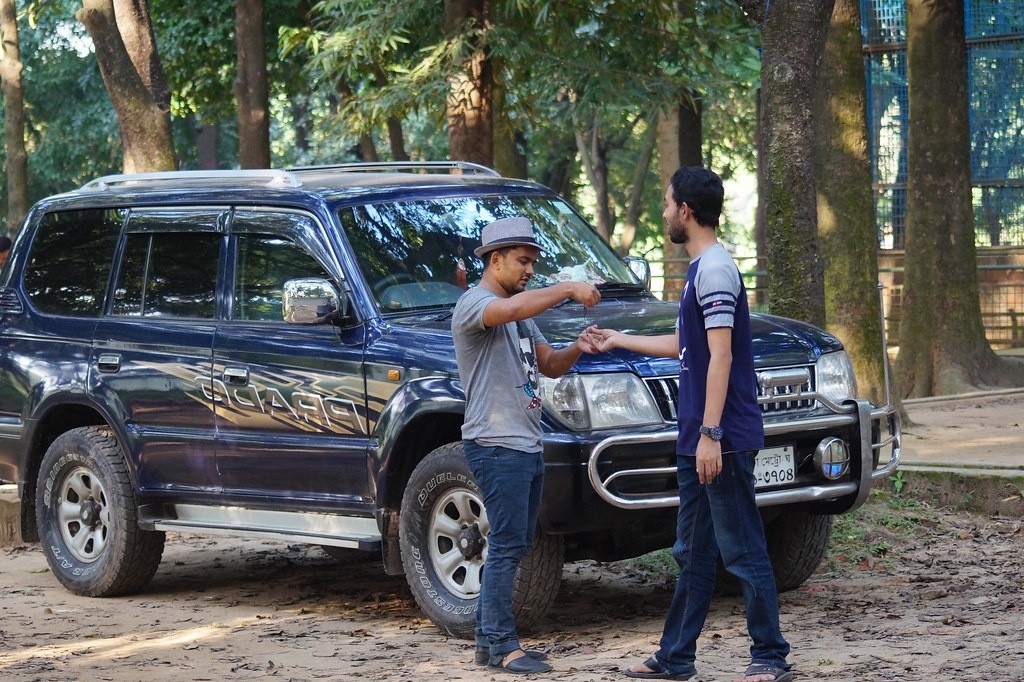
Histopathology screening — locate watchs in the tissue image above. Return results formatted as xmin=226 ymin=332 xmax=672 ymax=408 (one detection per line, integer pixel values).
xmin=699 ymin=426 xmax=723 ymax=441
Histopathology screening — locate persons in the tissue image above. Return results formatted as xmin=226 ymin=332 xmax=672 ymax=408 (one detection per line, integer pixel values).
xmin=450 ymin=218 xmax=600 ymax=676
xmin=578 ymin=168 xmax=794 ymax=682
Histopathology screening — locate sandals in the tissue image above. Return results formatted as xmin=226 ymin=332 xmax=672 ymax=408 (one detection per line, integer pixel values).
xmin=625 ymin=653 xmax=794 ymax=682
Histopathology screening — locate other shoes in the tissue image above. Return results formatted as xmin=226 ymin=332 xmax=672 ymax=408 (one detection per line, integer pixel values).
xmin=474 ymin=645 xmax=553 ymax=674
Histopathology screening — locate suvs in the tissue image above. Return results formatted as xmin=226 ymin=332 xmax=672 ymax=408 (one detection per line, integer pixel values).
xmin=0 ymin=158 xmax=903 ymax=641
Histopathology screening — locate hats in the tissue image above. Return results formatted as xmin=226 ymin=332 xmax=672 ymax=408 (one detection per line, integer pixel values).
xmin=474 ymin=217 xmax=547 ymax=259
